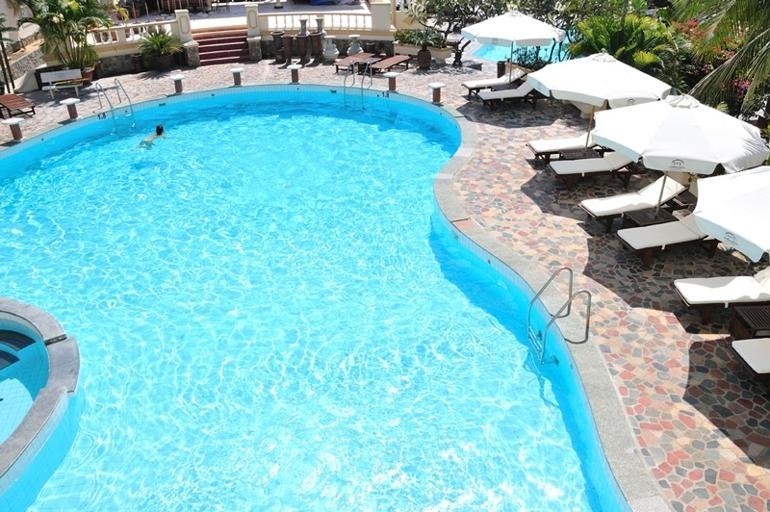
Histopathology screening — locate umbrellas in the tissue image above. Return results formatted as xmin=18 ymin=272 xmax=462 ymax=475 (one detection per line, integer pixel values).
xmin=692 ymin=165 xmax=770 ymax=263
xmin=461 ymin=7 xmax=566 ymax=87
xmin=590 ymin=91 xmax=769 ymax=220
xmin=526 ymin=49 xmax=672 ymax=150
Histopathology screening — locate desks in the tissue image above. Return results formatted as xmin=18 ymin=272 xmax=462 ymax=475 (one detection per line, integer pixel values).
xmin=627 ymin=208 xmax=677 ymax=228
xmin=558 ymin=147 xmax=593 ymax=162
xmin=731 ymin=302 xmax=770 ymax=337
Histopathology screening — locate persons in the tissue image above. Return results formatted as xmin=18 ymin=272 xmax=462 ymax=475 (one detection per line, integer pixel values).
xmin=144 ymin=125 xmax=163 ymax=142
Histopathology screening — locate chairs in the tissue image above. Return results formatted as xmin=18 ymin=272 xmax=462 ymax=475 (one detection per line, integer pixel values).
xmin=575 ymin=175 xmax=689 ymax=234
xmin=526 ymin=131 xmax=598 ymax=166
xmin=1 ymin=67 xmax=84 ymax=119
xmin=333 ymin=51 xmax=413 ymax=76
xmin=672 ymin=265 xmax=769 ymax=313
xmin=615 ymin=213 xmax=719 ymax=271
xmin=728 ymin=334 xmax=770 ymax=380
xmin=464 ymin=69 xmax=537 ymax=115
xmin=546 ymin=152 xmax=642 ymax=199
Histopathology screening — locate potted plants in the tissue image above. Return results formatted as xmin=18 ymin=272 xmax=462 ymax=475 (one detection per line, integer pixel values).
xmin=412 ymin=28 xmax=441 ymax=70
xmin=37 ymin=8 xmax=107 ymax=87
xmin=140 ymin=30 xmax=181 ymax=71
